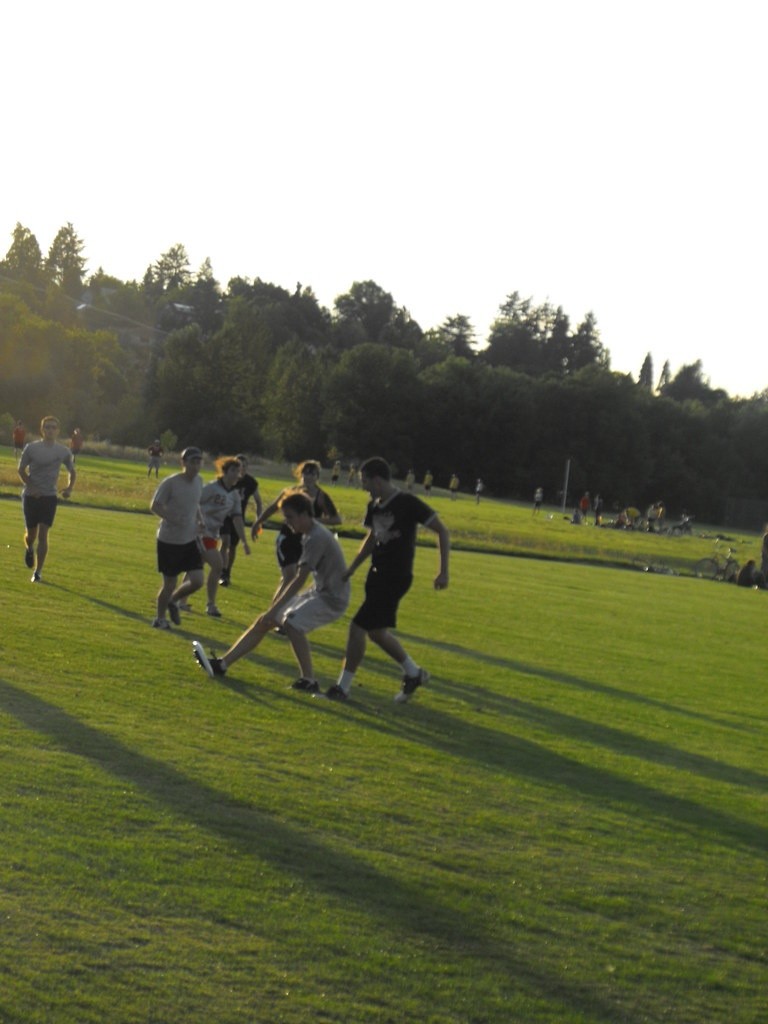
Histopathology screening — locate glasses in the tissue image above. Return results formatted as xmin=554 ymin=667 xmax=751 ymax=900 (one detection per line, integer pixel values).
xmin=46 ymin=425 xmax=57 ymax=430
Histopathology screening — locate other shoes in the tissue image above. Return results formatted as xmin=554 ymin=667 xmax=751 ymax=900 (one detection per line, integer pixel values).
xmin=177 ymin=601 xmax=192 ymax=611
xmin=192 ymin=641 xmax=228 ymax=679
xmin=31 ymin=571 xmax=41 ymax=583
xmin=287 ymin=678 xmax=321 ymax=693
xmin=312 ymin=685 xmax=354 ymax=702
xmin=206 ymin=603 xmax=222 ymax=617
xmin=272 ymin=626 xmax=286 ymax=635
xmin=218 ymin=573 xmax=229 ymax=587
xmin=167 ymin=602 xmax=181 ymax=625
xmin=393 ymin=668 xmax=428 ymax=702
xmin=152 ymin=618 xmax=173 ymax=631
xmin=24 ymin=548 xmax=34 ymax=568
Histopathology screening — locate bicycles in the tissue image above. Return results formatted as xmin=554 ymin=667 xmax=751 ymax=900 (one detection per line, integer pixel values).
xmin=693 ymin=537 xmax=741 ymax=581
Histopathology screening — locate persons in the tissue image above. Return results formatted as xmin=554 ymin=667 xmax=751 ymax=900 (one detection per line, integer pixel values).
xmin=13 ymin=421 xmax=26 ymax=458
xmin=194 ymin=489 xmax=350 ymax=692
xmin=148 ymin=440 xmax=165 ymax=479
xmin=533 ymin=488 xmax=693 ymax=538
xmin=72 ymin=419 xmax=88 ymax=466
xmin=17 ymin=415 xmax=76 ymax=582
xmin=321 ymin=457 xmax=448 ymax=705
xmin=737 ymin=560 xmax=766 ymax=588
xmin=404 ymin=467 xmax=486 ymax=504
xmin=330 ymin=460 xmax=360 ymax=487
xmin=760 ymin=527 xmax=768 ymax=588
xmin=152 ymin=447 xmax=263 ymax=631
xmin=251 ymin=461 xmax=343 ymax=635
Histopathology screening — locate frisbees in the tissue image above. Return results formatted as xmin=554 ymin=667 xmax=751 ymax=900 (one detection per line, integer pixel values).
xmin=193 ymin=641 xmax=214 ymax=677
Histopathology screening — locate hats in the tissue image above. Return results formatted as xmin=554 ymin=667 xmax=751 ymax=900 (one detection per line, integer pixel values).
xmin=181 ymin=446 xmax=202 ymax=461
